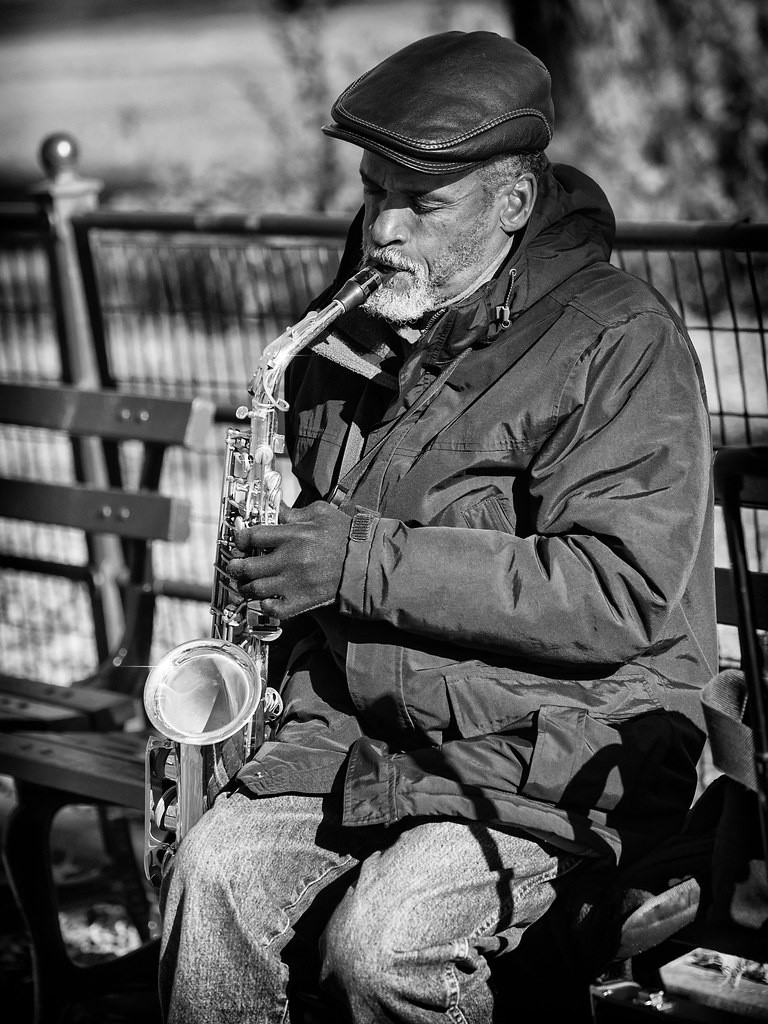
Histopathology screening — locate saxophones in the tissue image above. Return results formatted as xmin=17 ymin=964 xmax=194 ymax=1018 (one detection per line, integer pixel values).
xmin=143 ymin=263 xmax=398 ymax=887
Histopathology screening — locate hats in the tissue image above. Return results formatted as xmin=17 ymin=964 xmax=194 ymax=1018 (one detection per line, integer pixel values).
xmin=321 ymin=29 xmax=555 ymax=175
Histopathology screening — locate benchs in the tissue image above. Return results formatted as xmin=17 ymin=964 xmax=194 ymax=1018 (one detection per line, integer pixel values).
xmin=1 ymin=380 xmax=768 ymax=1023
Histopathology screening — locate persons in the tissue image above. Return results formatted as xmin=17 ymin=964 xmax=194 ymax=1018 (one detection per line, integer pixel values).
xmin=153 ymin=30 xmax=719 ymax=1024
